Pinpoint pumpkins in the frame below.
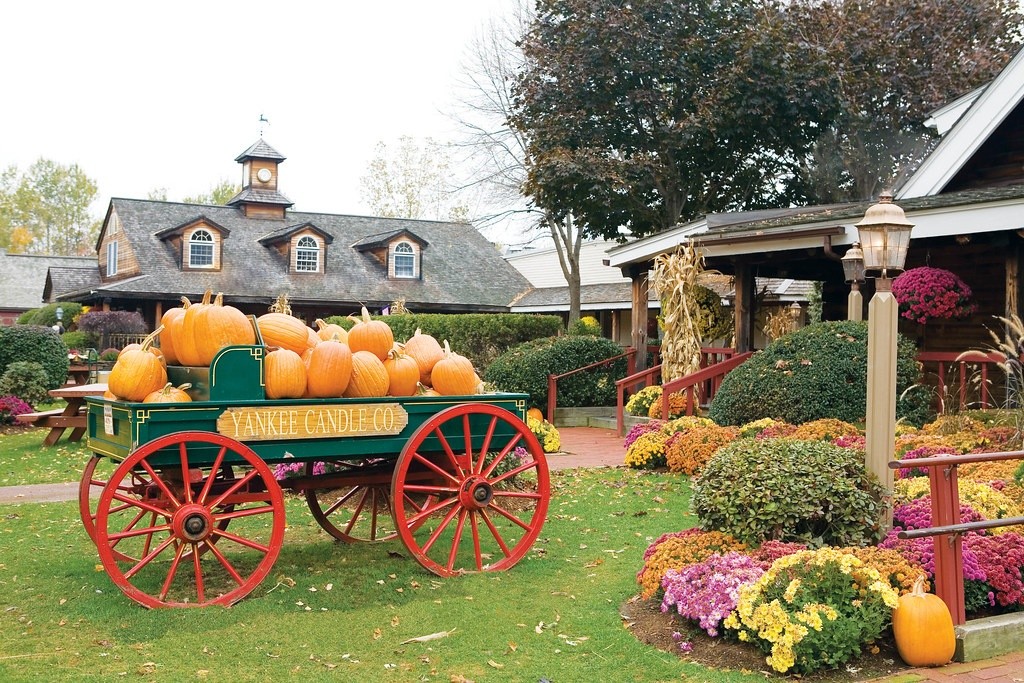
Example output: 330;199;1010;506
103;289;484;404
526;406;544;423
892;575;955;666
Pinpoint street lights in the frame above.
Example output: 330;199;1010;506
854;190;916;547
789;300;802;331
55;306;66;334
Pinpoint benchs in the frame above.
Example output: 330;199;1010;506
17;407;87;421
68;365;100;371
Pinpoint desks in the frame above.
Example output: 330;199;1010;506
71;360;105;384
32;384;110;448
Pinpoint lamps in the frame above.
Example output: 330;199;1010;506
55;306;65;321
853;192;914;292
840;241;867;285
789;300;802;322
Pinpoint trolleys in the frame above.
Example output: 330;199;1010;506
78;394;551;612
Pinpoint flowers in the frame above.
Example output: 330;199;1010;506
892;266;979;324
